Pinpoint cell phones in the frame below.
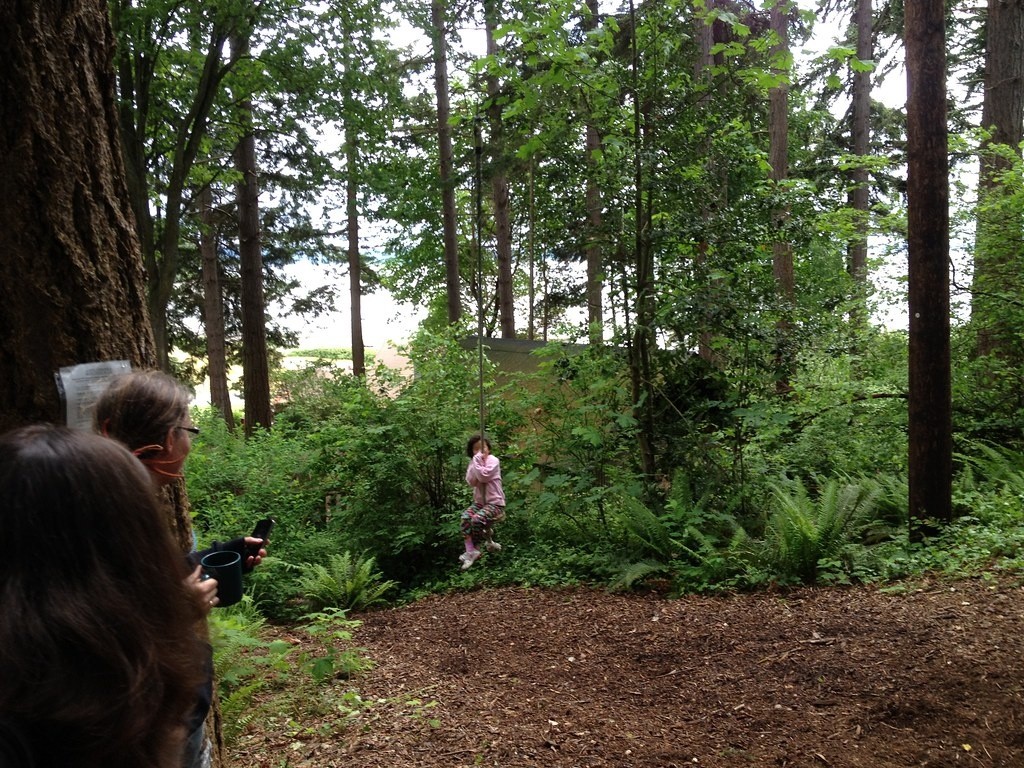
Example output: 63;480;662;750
245;519;275;561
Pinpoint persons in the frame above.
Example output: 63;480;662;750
90;368;271;768
459;436;506;569
0;426;209;768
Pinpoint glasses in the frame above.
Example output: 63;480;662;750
178;425;199;435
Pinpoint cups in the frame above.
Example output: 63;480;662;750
201;551;243;607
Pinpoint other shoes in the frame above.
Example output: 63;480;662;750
459;549;482;570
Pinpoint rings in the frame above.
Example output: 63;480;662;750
209;600;215;608
